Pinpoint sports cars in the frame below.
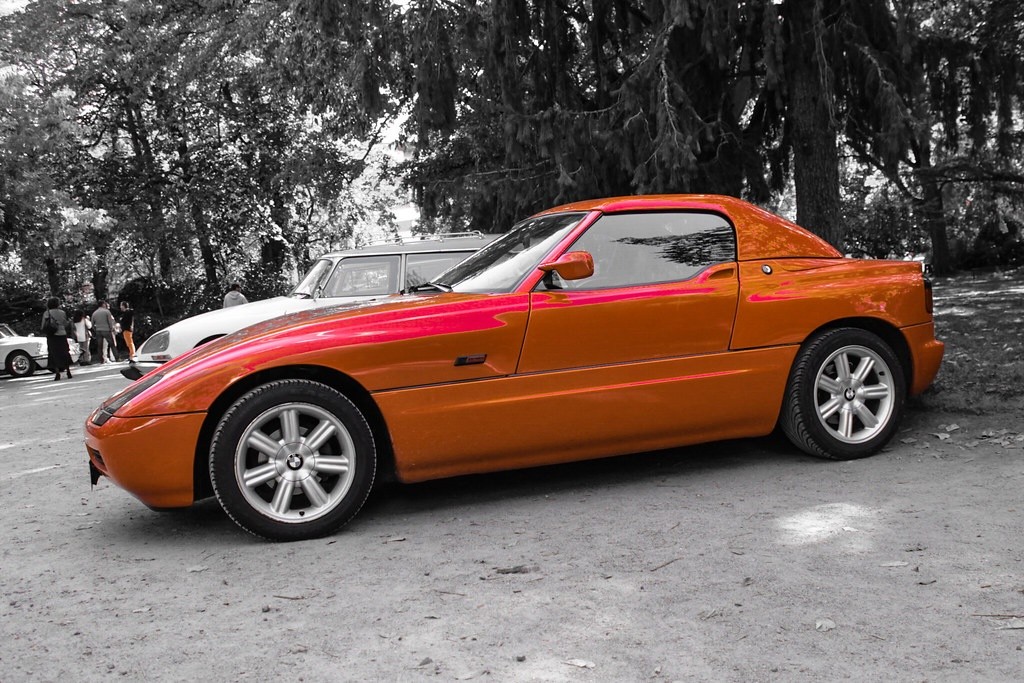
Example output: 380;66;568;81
83;190;945;543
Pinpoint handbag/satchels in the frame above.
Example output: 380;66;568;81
43;310;58;337
85;329;92;340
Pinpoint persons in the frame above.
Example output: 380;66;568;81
72;309;93;366
223;283;249;308
41;297;74;381
91;298;136;364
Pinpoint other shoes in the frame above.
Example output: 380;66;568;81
54;374;60;380
101;359;107;364
87;362;92;365
68;374;72;378
80;362;87;366
117;358;122;362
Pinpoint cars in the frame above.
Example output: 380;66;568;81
0;323;84;378
120;230;544;381
